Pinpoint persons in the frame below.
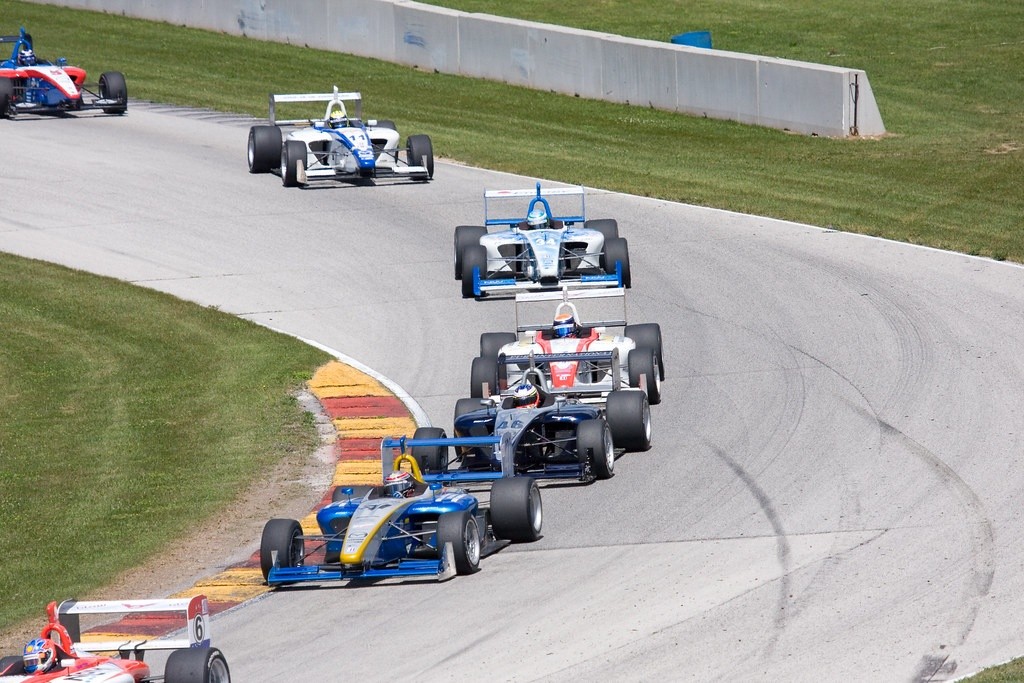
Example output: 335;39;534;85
526;209;552;230
552;313;578;338
20;636;59;675
513;384;540;410
18;48;40;67
382;470;421;498
328;111;352;128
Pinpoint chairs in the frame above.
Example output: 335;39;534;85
527;197;553;220
41;623;79;659
11;39;32;62
521;367;550;395
555;302;581;326
324;100;348;120
392;454;425;484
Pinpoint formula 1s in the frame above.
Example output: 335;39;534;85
0;27;128;117
453;180;632;298
411;349;652;481
247;85;434;187
470;285;665;405
260;433;543;587
0;594;231;683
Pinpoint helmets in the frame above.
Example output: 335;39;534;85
553;313;577;338
19;49;36;66
383;470;415;497
328;111;347;128
23;638;57;676
526;210;549;230
512;385;540;408
